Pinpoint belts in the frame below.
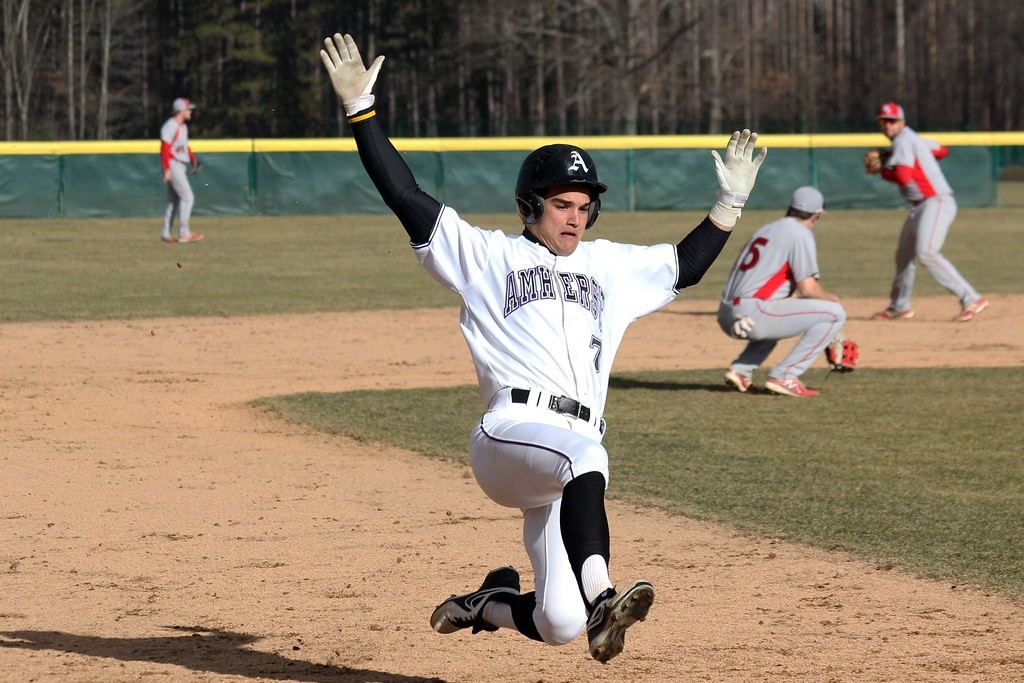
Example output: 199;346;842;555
511;389;604;435
913;197;929;205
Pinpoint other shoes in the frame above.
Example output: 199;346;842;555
871;307;916;320
161;234;178;243
953;297;989;321
177;232;204;243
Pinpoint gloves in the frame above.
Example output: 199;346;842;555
320;33;385;117
709;129;767;227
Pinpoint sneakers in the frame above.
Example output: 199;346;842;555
586;580;654;664
430;565;520;634
724;369;760;394
764;377;820;397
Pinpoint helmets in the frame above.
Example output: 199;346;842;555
515;144;608;230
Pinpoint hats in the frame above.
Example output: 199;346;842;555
172;97;197;112
790;186;825;213
874;102;905;120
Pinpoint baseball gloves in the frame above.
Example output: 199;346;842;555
189;160;204;174
863;148;886;174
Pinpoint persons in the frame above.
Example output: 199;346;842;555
863;100;989;322
160;98;204;243
318;32;769;666
717;186;859;401
